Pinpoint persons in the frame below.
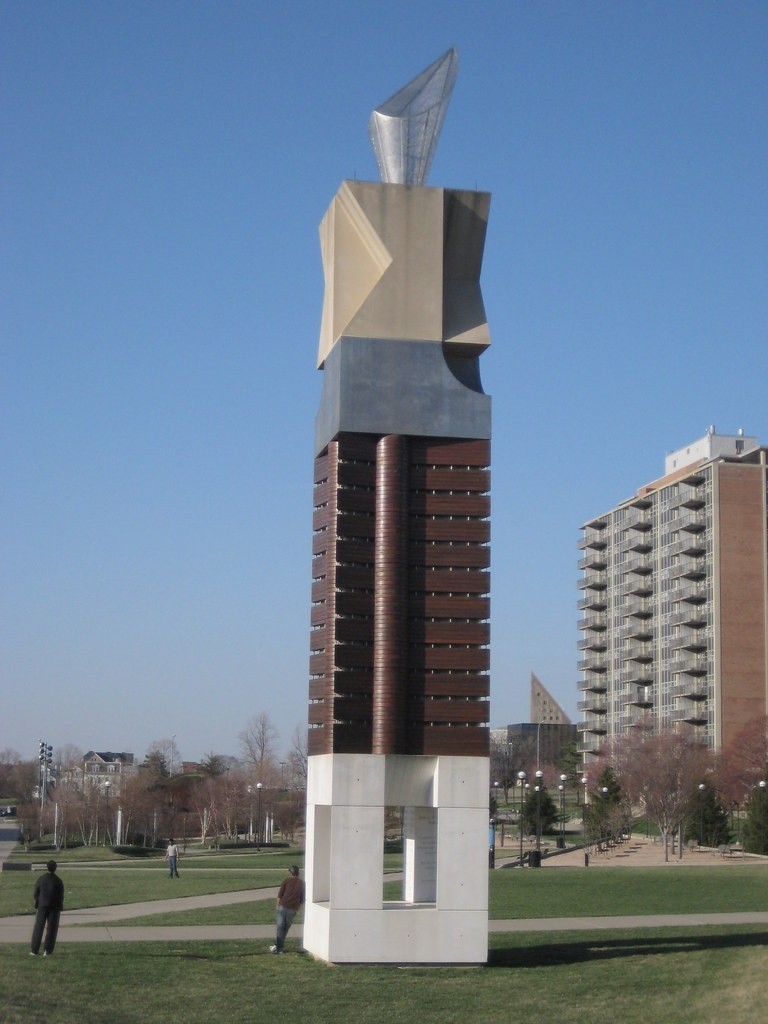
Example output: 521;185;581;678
29;860;64;958
270;865;305;954
164;839;180;878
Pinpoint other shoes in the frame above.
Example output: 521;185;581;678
29;952;39;956
44;951;50;956
269;942;283;955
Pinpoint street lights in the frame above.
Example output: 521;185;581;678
759;781;767;855
494;782;500;830
37;738;41;809
535;771;543;850
103;781;111;847
525;784;531;802
582;778;588;845
171;734;177;805
601;786;608;849
560;775;567;849
699;784;706;847
257;783;263;851
518;772;526;867
559;785;563;839
39;741;53;838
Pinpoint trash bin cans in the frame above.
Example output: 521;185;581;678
556;837;564;848
529;850;541;867
